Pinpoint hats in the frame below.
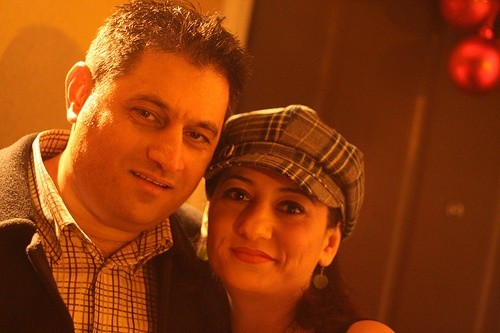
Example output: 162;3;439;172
203;104;365;240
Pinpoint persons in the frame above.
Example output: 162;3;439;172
0;1;253;333
199;105;393;333
